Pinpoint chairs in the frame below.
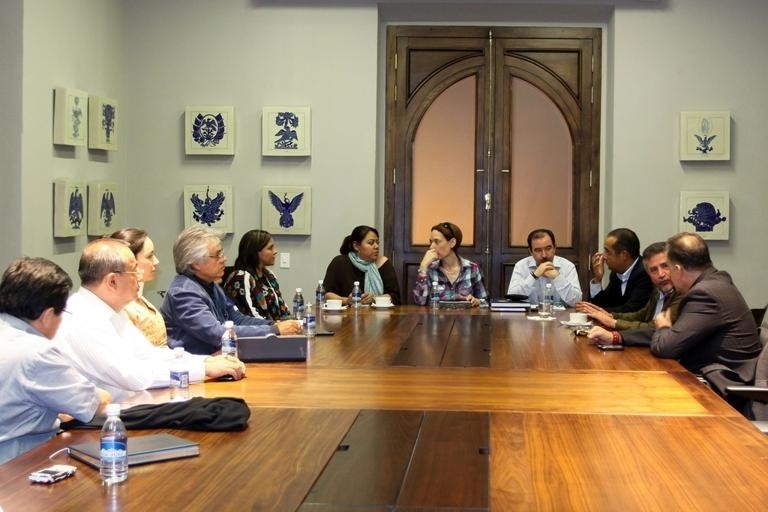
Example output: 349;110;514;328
217;265;236;289
158;291;168;297
698;302;768;436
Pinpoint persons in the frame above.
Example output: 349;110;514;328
575;242;683;331
413;222;487;306
160;224;303;355
52;240;246;403
506;229;584;308
100;230;168;404
1;258;112;464
588;227;655;312
324;226;402;306
586;232;764;423
225;228;291;321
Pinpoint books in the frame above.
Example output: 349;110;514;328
489;297;530;308
490;305;527;312
68;428;200;473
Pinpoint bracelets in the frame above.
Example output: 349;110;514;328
612;331;620;345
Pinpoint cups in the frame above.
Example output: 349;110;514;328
324;298;343;309
569;312;587;324
537;300;550;318
375;297;390;305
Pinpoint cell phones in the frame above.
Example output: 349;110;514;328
574;329;589;336
597;344;624;351
218;375;233;381
29;465;77;484
315;331;335;336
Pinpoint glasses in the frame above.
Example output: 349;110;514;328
112;267;144;281
198;248;225;259
440;220;456;234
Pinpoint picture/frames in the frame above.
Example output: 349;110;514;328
261;103;313;159
260;185;314;237
677;189;733;243
182;184;234;235
677;109;731;164
183;104;234;159
53;85;124;242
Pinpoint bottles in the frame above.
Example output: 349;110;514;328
98;403;129;483
351;280;361;312
168;346;190;401
544;284;554;317
292;280;327;339
221;320;238;357
430;282;440;310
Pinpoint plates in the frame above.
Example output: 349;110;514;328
566;322;591;327
371;305;395;309
322;306;347;311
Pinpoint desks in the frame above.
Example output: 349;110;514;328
0;301;768;511
